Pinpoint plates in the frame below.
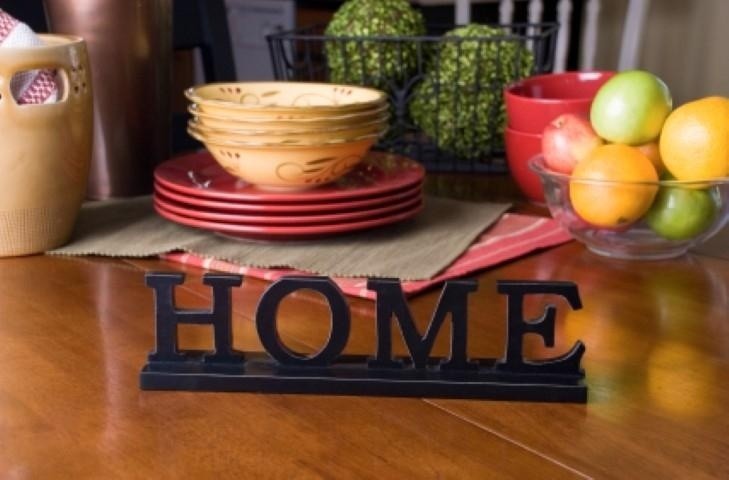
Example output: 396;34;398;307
154;151;430;239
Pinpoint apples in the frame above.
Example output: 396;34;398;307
542;112;604;176
635;139;667;177
590;71;674;144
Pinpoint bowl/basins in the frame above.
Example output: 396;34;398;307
182;77;391;190
505;69;624;130
499;131;590;202
534;149;728;261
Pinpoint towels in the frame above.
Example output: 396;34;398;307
154;212;578;307
53;196;513;283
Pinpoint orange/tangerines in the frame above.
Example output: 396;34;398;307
637;255;712;337
646;171;716;241
